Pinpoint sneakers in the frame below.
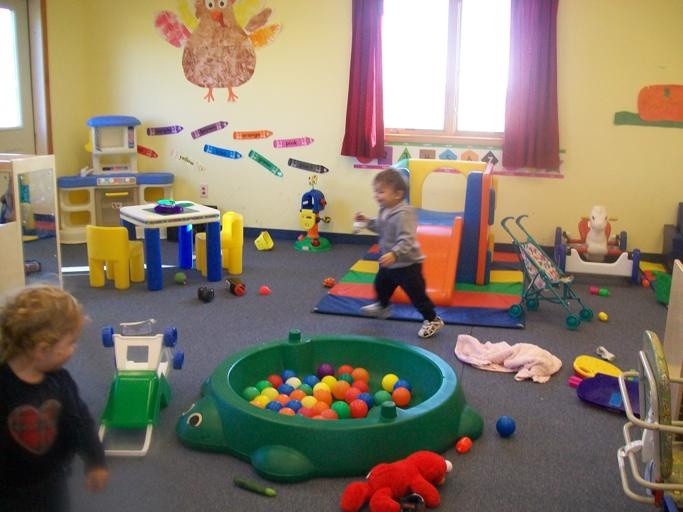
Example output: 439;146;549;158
359;300;392;320
417;315;445;338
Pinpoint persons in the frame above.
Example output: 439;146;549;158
353;169;445;338
0;285;108;512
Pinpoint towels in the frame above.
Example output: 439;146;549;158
455;334;562;384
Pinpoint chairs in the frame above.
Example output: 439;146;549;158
196;212;243;275
87;225;144;289
618;329;683;512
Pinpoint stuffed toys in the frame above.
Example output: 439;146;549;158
341;449;453;511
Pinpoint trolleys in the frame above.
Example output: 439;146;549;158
500;212;595;331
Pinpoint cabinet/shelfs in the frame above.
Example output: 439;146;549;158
0;153;58;292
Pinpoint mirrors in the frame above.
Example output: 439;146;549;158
18;169;60;285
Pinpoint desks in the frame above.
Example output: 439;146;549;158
119;200;222;291
